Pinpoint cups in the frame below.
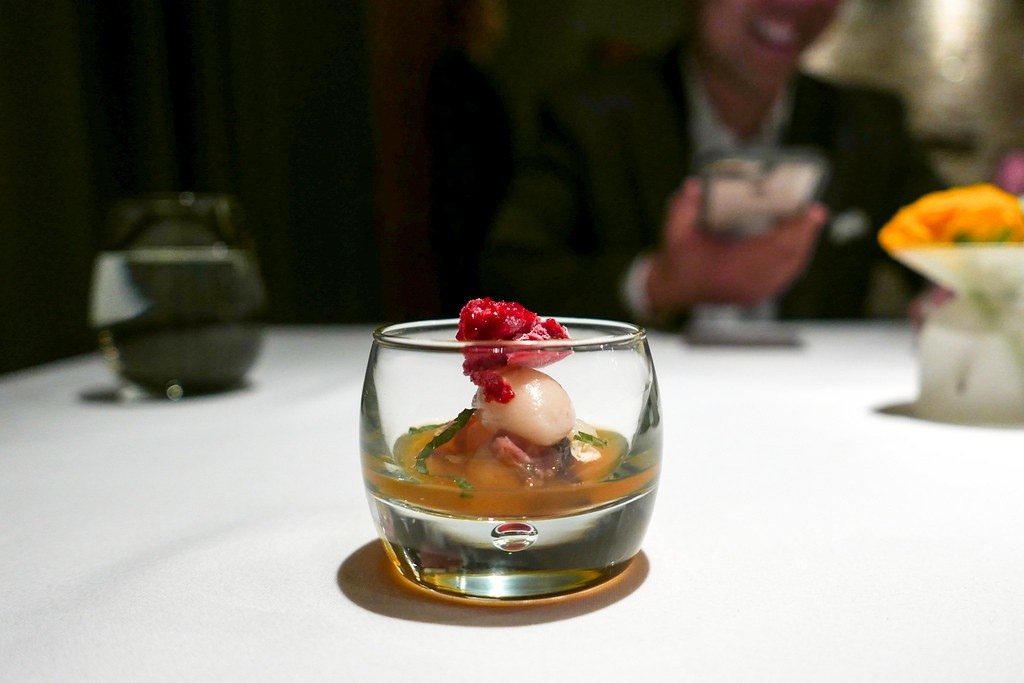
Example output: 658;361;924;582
361;315;661;604
91;198;270;395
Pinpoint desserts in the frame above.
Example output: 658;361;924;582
449;297;576;445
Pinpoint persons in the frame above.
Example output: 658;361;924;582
480;0;949;334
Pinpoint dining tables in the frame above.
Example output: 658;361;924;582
0;321;1024;683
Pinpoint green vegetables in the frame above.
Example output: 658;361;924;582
407;408;606;499
